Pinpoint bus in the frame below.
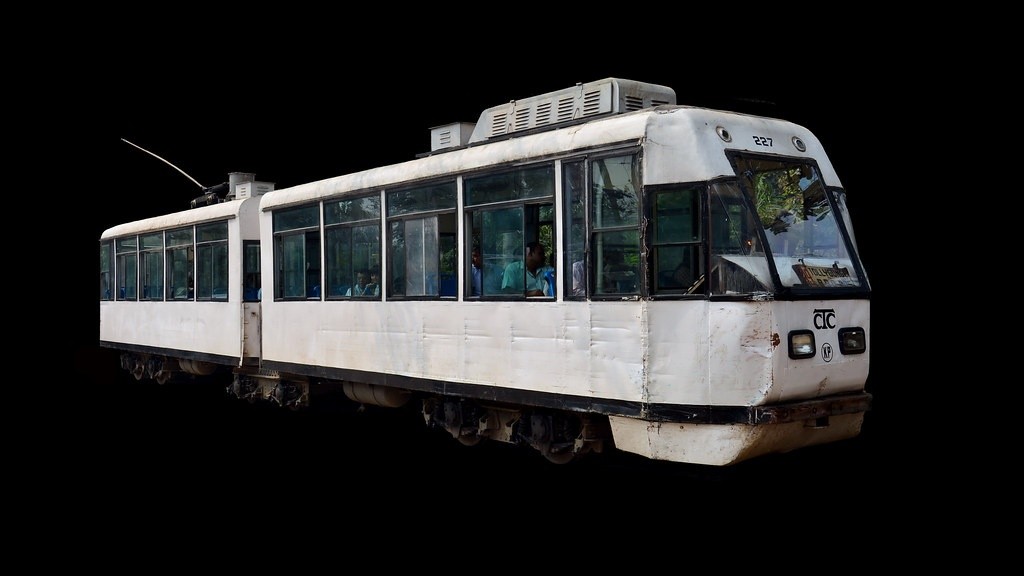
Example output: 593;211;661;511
96;76;874;467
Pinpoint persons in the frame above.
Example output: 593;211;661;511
283;228;699;296
772;213;800;257
104;253;225;299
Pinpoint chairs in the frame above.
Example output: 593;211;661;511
213;262;641;300
